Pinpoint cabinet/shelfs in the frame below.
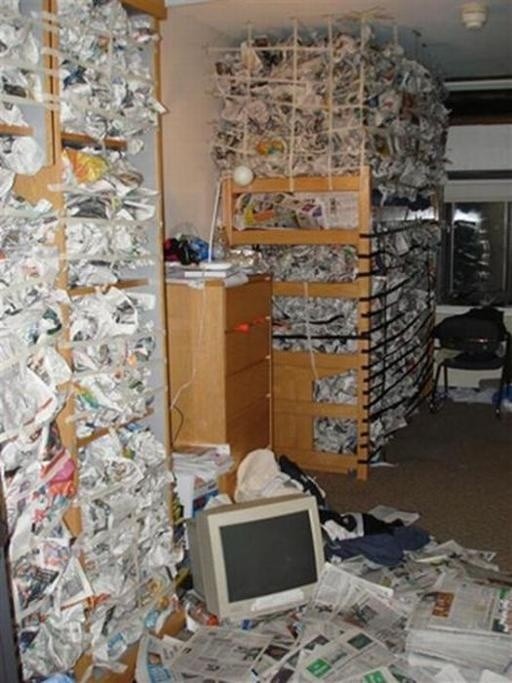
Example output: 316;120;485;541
0;0;189;683
165;270;274;502
221;163;446;482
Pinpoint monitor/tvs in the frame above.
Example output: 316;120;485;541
185;495;327;622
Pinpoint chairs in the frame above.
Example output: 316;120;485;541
428;306;511;422
